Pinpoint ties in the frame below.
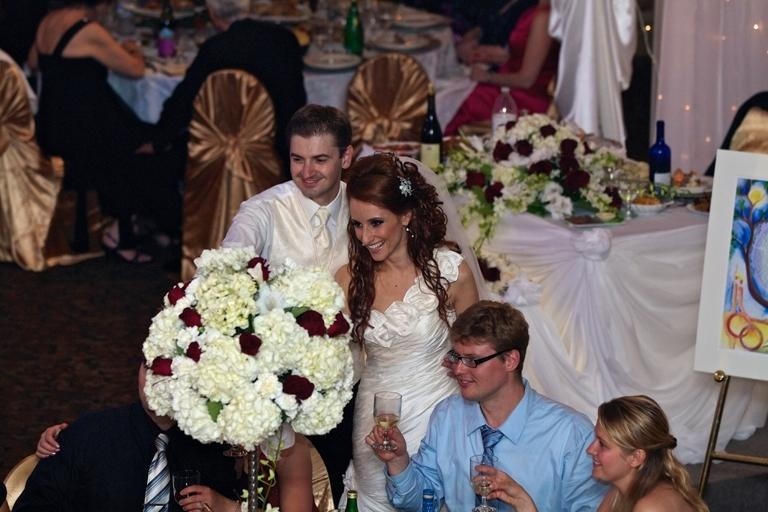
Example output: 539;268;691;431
144;432;171;511
314;208;332;255
475;425;503;511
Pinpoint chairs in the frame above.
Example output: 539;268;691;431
339;49;428;164
1;53;109;274
173;64;288;279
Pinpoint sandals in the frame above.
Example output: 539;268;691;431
101;228;154;264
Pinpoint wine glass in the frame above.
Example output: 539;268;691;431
170;469;201;510
468;454;502;512
374;390;403;451
614;171;643;220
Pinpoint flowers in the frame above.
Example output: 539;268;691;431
132;244;362;459
436;107;657;295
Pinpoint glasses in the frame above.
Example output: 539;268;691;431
445;349;513;368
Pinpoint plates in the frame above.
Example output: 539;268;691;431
300;53;362;71
370;30;432;52
682;200;711;215
244;4;308;21
390;11;444;29
119;1;195;18
625;202;673;215
662;175;716;196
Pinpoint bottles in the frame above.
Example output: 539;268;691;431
345;2;364;55
421;79;444;172
344;491;359;511
490;86;519;147
159;2;177;57
648;119;671;187
421;488;435;511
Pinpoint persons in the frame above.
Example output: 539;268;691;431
2;2;709;511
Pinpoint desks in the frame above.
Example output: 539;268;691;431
82;3;456;177
420;169;768;480
